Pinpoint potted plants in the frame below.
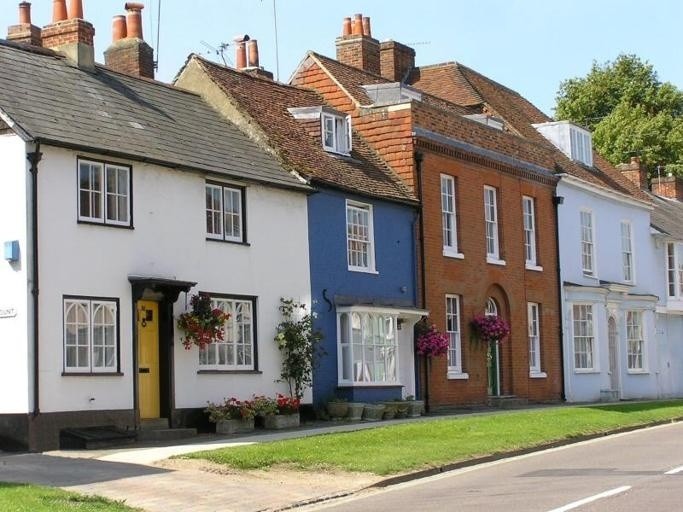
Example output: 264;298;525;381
325;395;424;422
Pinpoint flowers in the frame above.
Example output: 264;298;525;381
412;317;450;361
202;391;302;423
172;291;231;351
271;294;327;413
468;314;511;343
